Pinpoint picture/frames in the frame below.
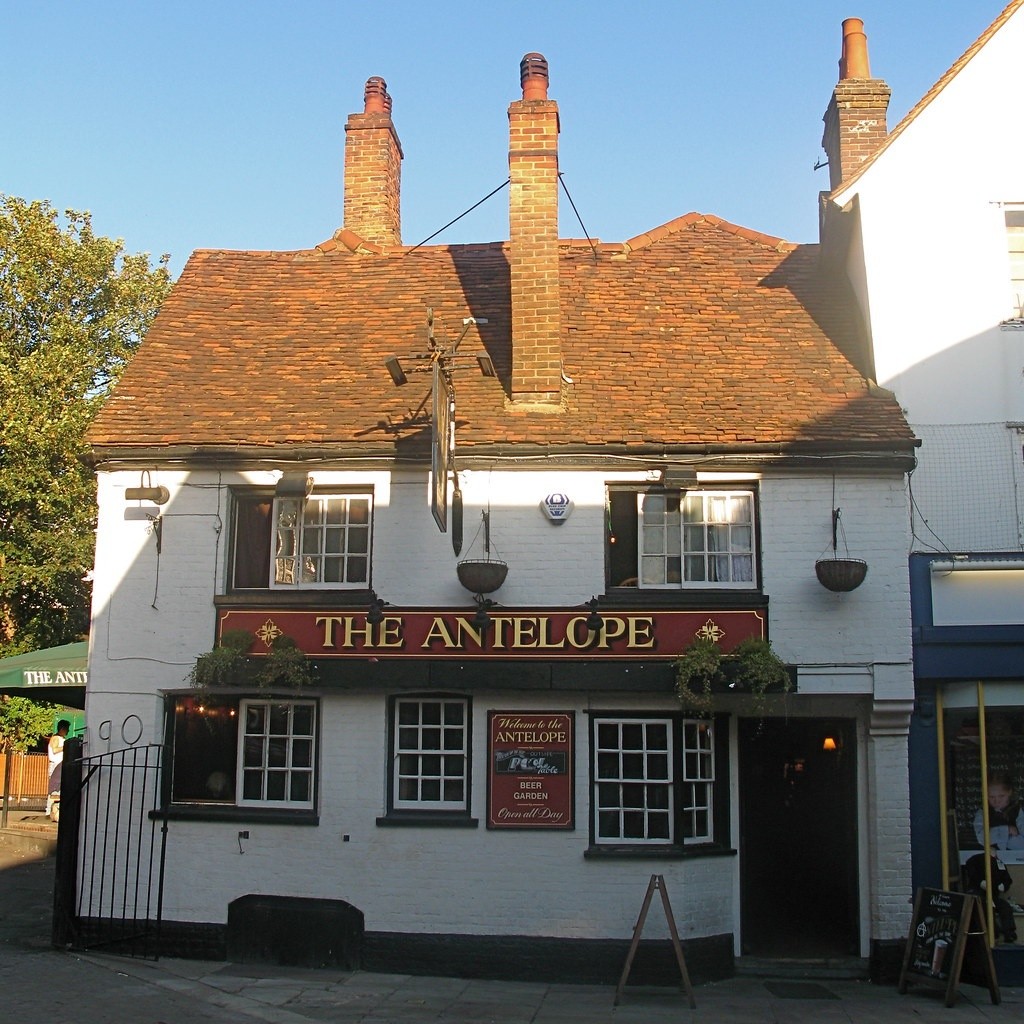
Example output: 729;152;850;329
487;709;577;830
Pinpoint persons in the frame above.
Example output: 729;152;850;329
974;775;1024;851
46;720;71;822
966;843;1018;946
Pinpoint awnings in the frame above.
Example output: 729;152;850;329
0;641;89;711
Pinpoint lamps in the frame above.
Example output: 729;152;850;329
125;469;170;505
367;590;390;623
662;465;700;491
585;595;604;630
275;470;314;500
474;593;498;629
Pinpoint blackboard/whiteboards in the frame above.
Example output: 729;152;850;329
903;887;974;991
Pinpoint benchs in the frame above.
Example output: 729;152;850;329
228;893;367;972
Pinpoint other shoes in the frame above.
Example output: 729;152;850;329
1013;905;1024;912
